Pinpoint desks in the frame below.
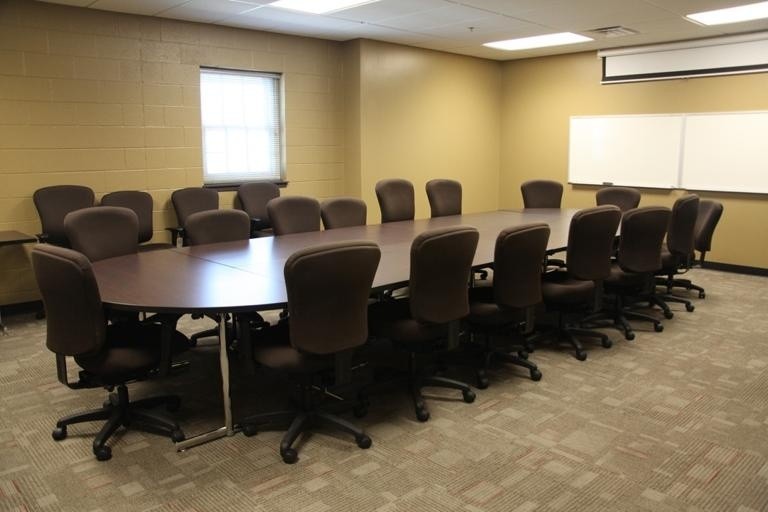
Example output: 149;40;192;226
91;208;624;452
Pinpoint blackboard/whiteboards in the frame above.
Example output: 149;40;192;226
679;111;768;193
566;113;685;190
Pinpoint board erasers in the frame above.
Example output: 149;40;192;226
603;182;613;185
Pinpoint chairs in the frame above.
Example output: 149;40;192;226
577;208;672;340
655;201;722;298
321;199;367;228
377;182;414;223
426;181;462;217
432;224;550;380
184;208;268;343
64;205;190;373
101;191;174;252
521;181;562;209
266;196;321;236
32;243;191;460
596;187;640;211
237;182;280;236
624;194;698;319
353;228;479;421
524;208;622;361
241;244;380;463
34;186;94;249
164;187;218;247
